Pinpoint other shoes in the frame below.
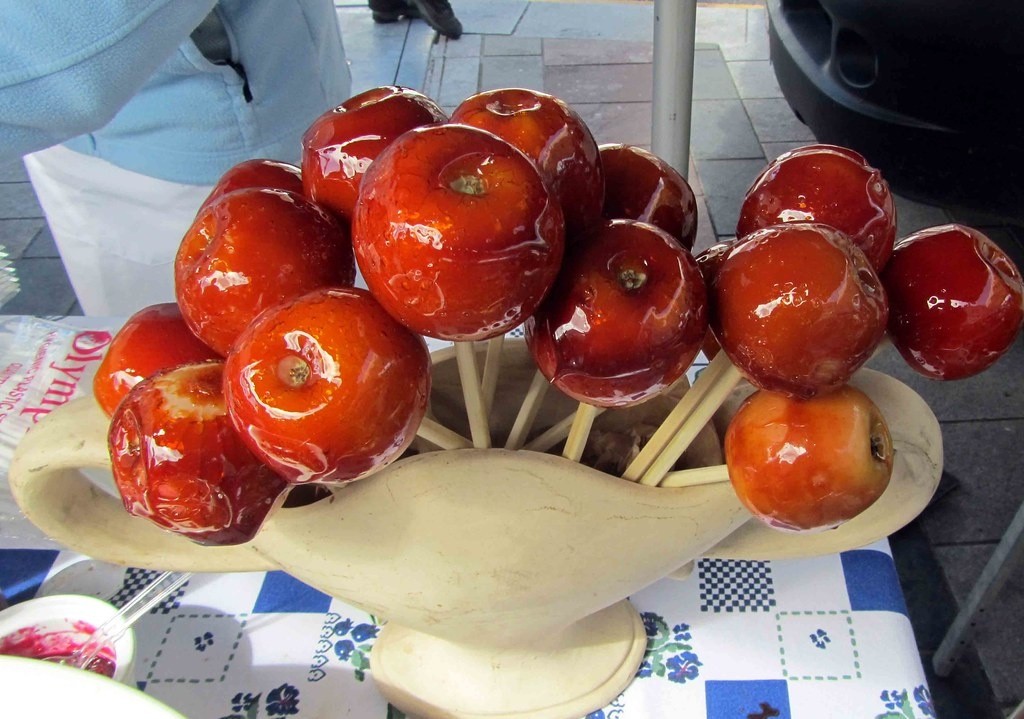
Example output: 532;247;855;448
368;0;421;23
413;0;462;40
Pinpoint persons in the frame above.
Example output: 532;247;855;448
0;0;355;320
369;0;462;40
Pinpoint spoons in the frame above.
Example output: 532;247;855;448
41;570;197;671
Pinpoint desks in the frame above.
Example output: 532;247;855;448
0;313;940;719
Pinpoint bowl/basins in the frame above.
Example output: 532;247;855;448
0;592;135;683
